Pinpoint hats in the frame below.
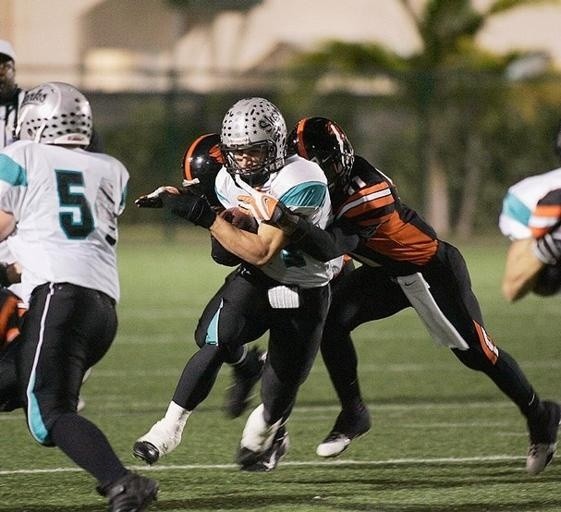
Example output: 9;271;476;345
0;37;15;62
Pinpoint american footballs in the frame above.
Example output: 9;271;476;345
220;207;258;233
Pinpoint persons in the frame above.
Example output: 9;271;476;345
499;167;561;304
0;38;26;147
134;98;344;470
0;81;159;512
210;117;561;474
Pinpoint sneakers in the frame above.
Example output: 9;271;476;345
133;418;186;462
522;397;560;475
239;425;290;473
236;401;279;467
225;350;269;420
315;405;372;457
99;469;161;512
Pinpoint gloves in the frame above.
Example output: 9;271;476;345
135;186;174;211
237;187;280;225
162;189;216;229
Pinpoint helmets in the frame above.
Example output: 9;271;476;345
14;81;94;149
219;93;288;178
181;131;224;191
286;115;356;191
